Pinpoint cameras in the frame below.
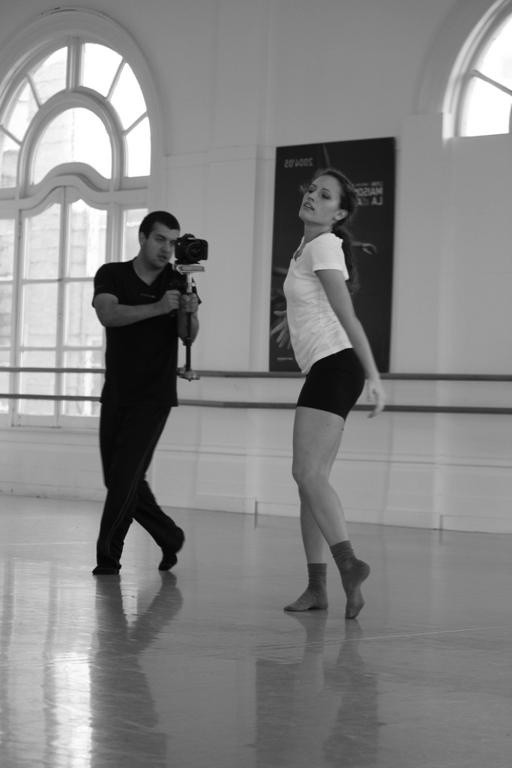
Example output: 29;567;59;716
174;233;209;263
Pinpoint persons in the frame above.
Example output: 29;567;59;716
267;168;386;621
251;604;380;768
87;563;184;768
91;209;204;576
